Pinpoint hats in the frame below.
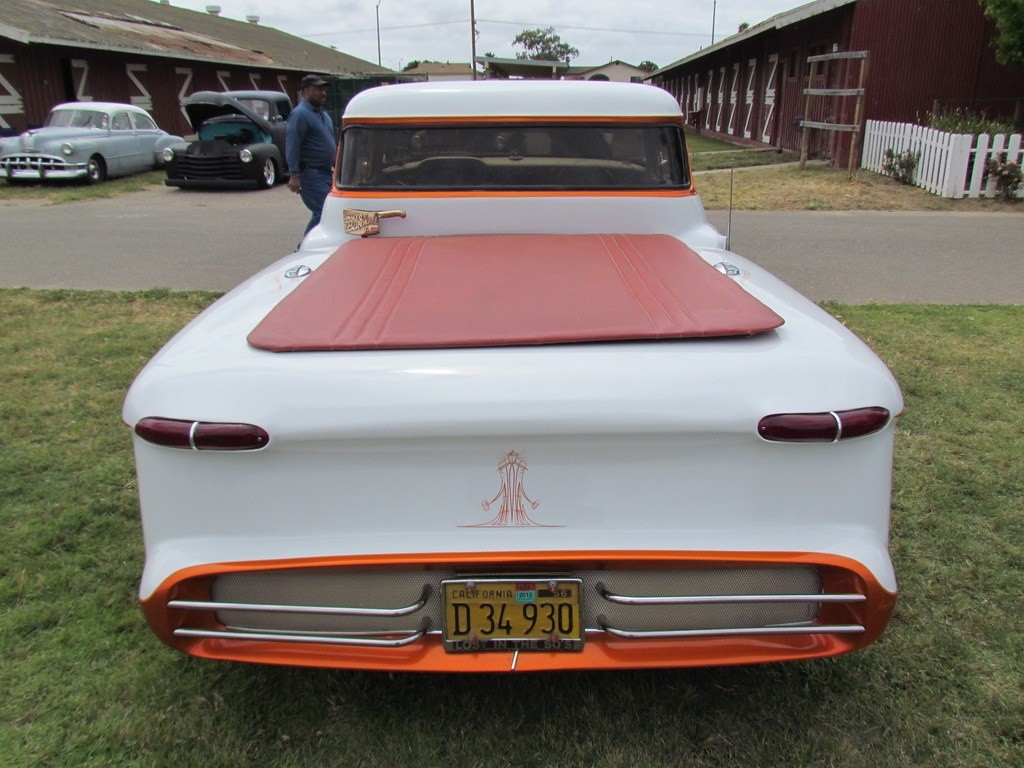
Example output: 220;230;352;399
300;74;330;90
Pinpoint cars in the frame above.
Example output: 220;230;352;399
163;87;294;189
0;102;184;184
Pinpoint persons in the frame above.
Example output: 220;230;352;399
285;74;338;254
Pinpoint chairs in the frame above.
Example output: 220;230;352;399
409;158;489;189
545;153;614;189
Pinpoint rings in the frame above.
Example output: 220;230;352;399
299;186;301;190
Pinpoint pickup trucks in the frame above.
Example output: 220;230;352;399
123;81;905;674
